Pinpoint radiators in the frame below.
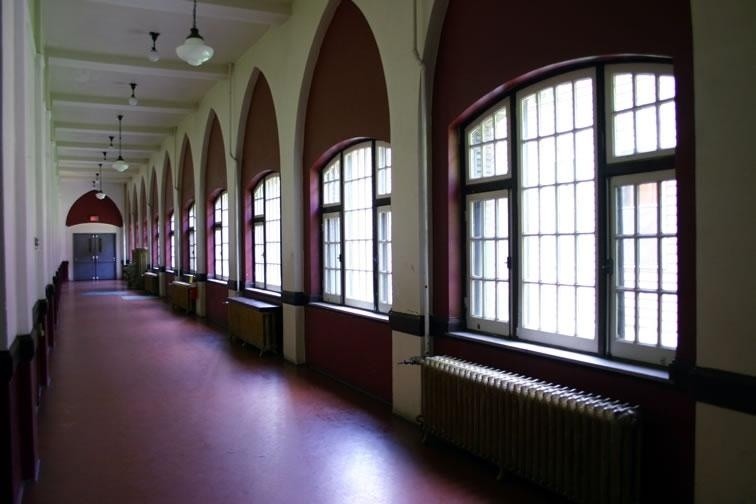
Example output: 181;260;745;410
419;355;640;504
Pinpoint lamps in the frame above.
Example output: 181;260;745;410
95;163;106;200
111;114;129;173
175;0;215;67
